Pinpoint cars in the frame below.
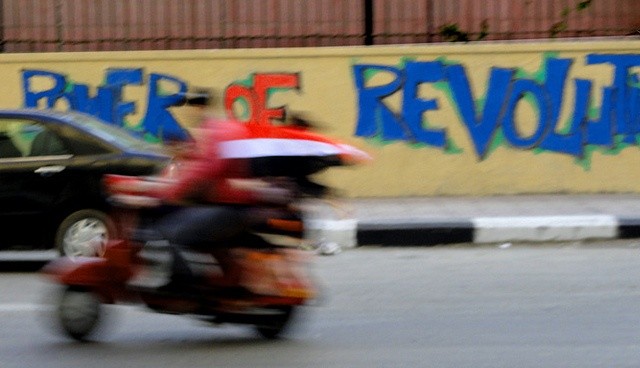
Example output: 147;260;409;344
0;107;182;266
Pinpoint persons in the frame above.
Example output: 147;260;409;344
125;86;267;287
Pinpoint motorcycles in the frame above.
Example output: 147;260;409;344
37;115;372;341
